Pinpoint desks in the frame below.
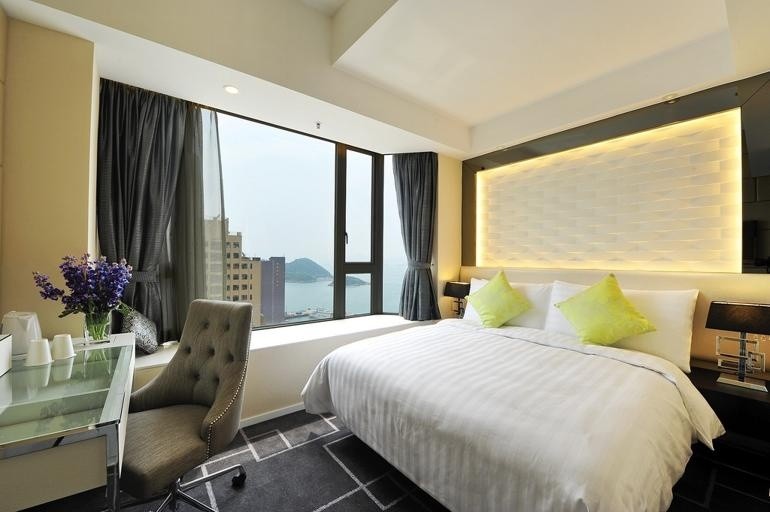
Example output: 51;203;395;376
0;331;137;511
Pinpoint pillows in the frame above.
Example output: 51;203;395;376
463;278;551;329
554;274;655;345
122;307;158;353
545;281;699;373
465;270;531;328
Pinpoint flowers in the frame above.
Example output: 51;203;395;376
32;250;133;318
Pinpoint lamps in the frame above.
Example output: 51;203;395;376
444;281;470;316
706;301;770;394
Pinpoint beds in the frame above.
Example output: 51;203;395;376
300;320;726;512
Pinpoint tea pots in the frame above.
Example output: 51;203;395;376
2;311;43;361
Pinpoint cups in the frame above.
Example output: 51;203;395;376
52;358;74;382
24;338;54;367
25;364;52;388
53;334;77;360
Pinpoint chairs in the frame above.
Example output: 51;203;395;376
121;299;254;512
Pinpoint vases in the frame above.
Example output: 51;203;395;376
84;311;111;343
